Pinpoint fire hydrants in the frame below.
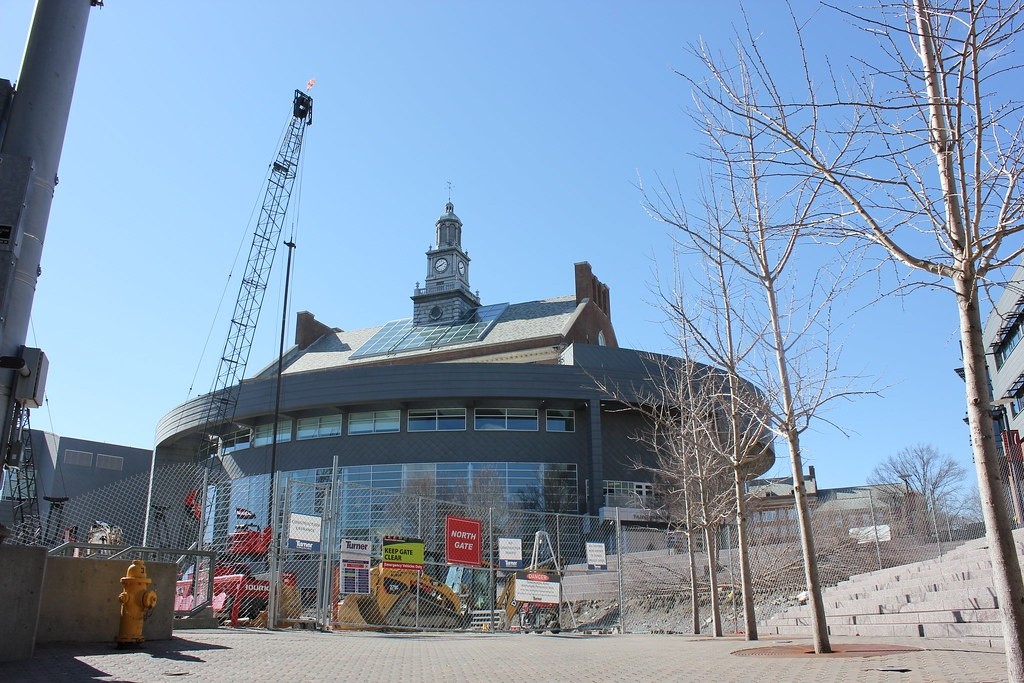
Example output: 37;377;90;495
112;556;159;649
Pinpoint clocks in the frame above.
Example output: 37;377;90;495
458;261;465;275
434;258;448;272
